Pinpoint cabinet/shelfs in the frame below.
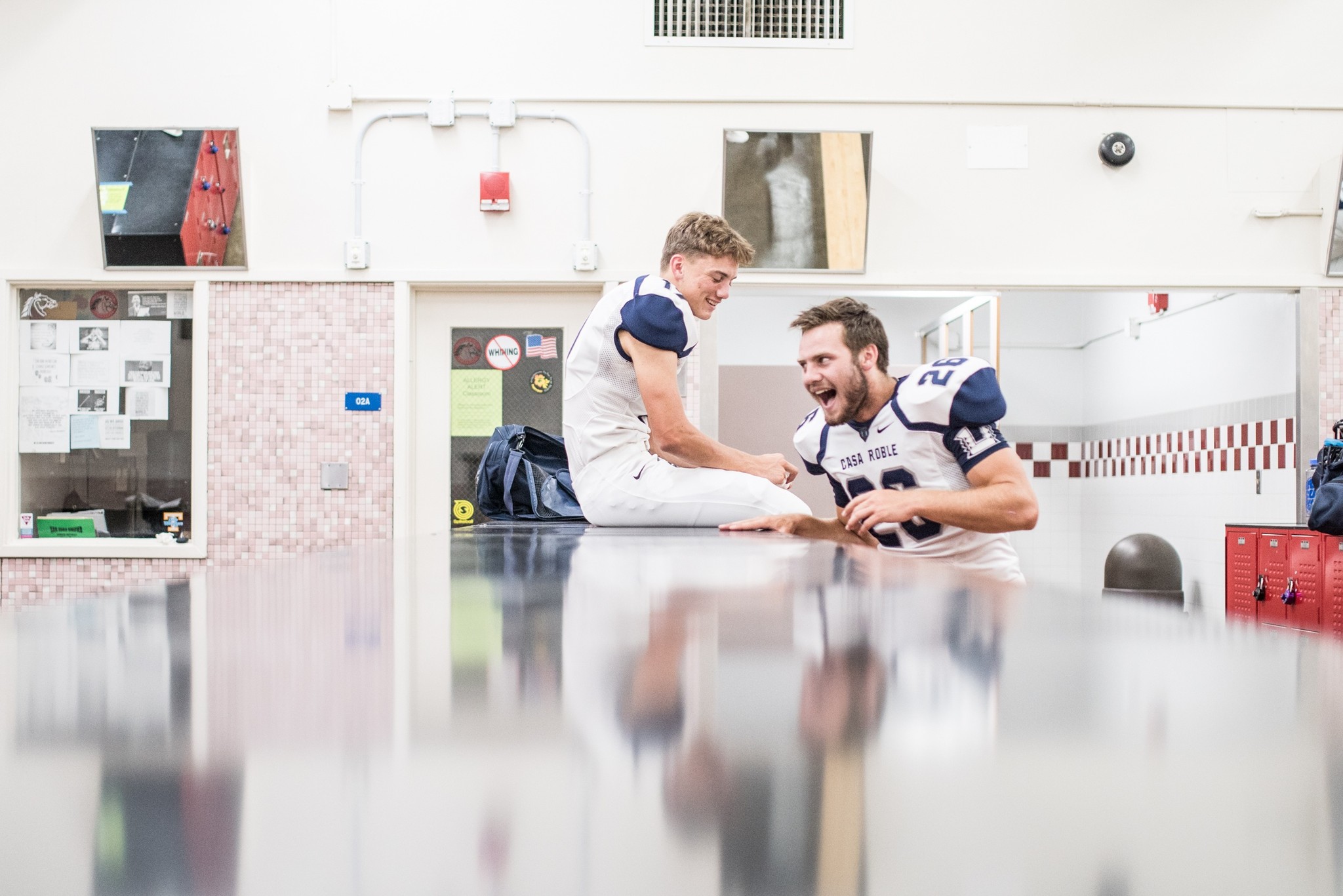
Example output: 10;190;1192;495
94;130;240;266
1223;523;1343;647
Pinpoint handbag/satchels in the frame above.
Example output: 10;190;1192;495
475;424;586;521
1308;460;1343;536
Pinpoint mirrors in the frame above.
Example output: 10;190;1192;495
721;128;873;274
91;127;248;272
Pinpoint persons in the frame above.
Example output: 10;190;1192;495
754;137;816;268
716;297;1039;583
80;328;108;351
138;361;152;371
561;529;1004;834
562;213;813;527
128;295;150;316
